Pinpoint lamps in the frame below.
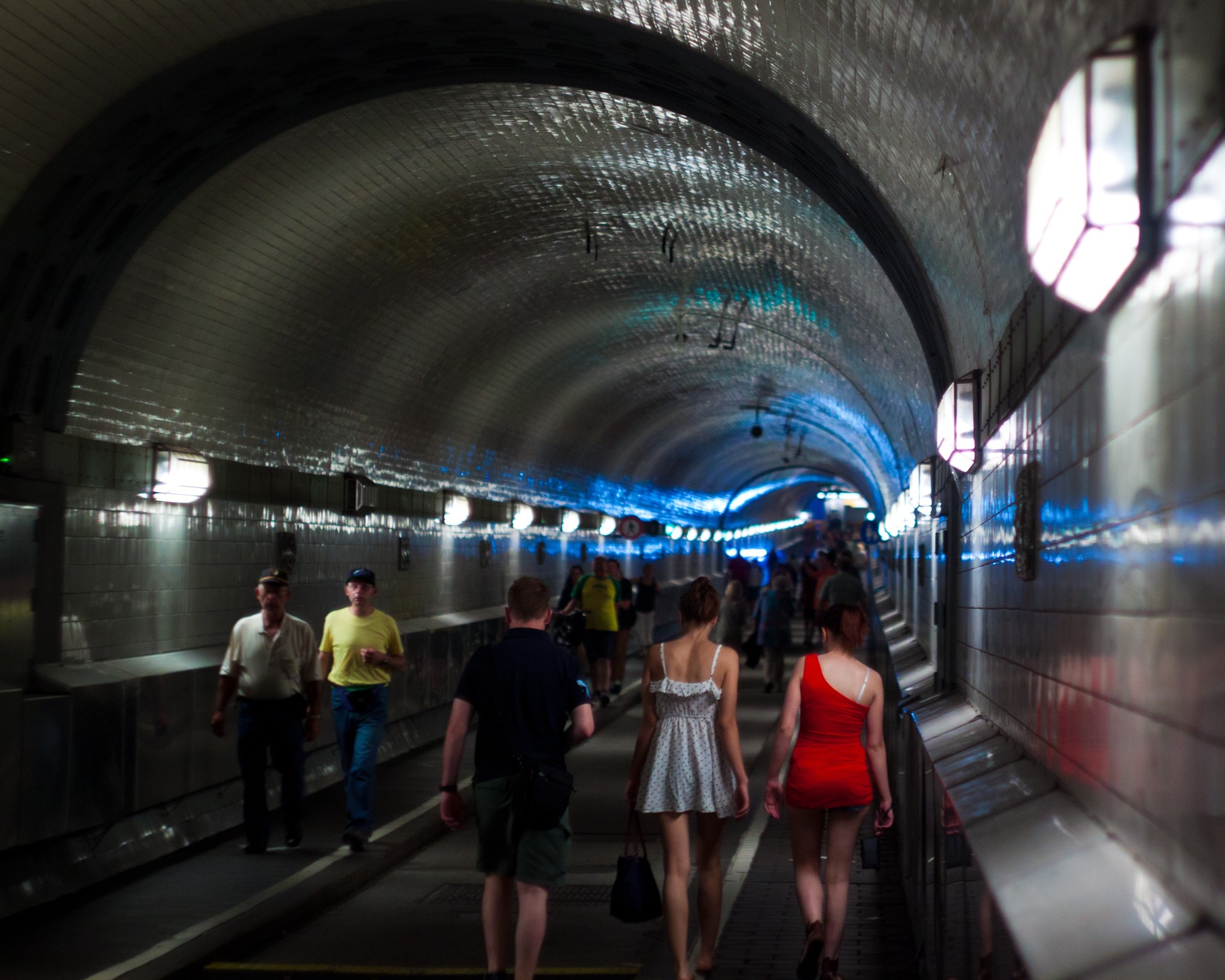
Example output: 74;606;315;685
151;442;213;505
343;472;379;517
1026;0;1158;315
909;455;937;517
935;369;982;475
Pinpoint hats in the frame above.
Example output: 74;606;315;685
258;569;290;588
347;568;377;585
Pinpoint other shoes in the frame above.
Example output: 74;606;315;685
342;828;368;852
285;817;302;847
245;830;267;854
600;691;611;707
795;928;822;980
610;676;623;695
765;681;776;693
818;957;844;980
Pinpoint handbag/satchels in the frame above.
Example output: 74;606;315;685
609;800;663;923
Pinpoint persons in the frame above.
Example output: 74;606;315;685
715;581;747;651
763;603;894;980
751;575;797;693
785;545;861;647
747;559;762;604
210;564;319;855
440;577;595;980
318;567;406;854
555;558;633;708
625;576;750;980
632;564;661;651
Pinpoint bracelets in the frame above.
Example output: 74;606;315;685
440;785;457;792
